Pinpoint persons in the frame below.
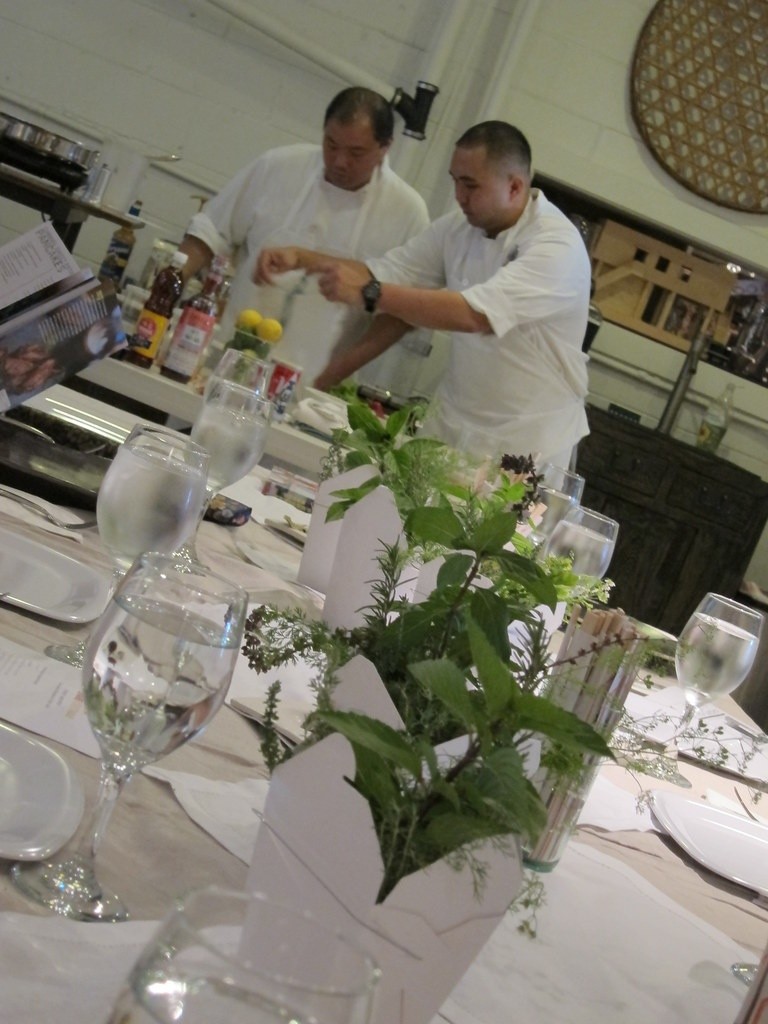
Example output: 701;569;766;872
249;120;594;497
171;85;430;391
0;316;123;398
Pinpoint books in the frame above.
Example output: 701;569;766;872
0;220;95;321
1;276;129;416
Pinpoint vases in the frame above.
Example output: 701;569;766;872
249;731;522;1024
332;650;540;780
319;483;420;637
297;457;387;594
411;555;565;653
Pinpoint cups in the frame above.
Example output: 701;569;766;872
224;329;272;358
515;463;584;545
267;357;305;414
106;888;379;1024
517;679;623;873
545;505;619;598
202;349;276;414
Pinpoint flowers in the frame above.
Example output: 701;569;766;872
318;394;615;618
243;551;721;744
242;619;767;941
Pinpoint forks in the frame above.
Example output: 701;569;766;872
0;489;97;531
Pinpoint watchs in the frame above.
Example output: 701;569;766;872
362;278;381;316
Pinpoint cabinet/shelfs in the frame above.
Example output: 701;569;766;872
577;403;768;637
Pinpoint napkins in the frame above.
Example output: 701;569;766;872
141;764;759;1024
0;912;244;1023
579;777;668;836
705;783;768;827
220;479;312;529
158;591;326;751
0;484;85;546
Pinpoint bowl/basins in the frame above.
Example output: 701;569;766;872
302;385;350;413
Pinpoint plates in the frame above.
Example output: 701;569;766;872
649;786;768;896
0;726;86;861
639;620;686;661
0;529;117;623
620;686;768;785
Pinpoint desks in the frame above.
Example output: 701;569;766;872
0;385;768;1024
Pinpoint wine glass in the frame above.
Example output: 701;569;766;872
732;963;760;986
10;551;249;923
42;425;211;664
175;382;273;572
660;592;763;747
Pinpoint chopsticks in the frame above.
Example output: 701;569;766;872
523;602;646;863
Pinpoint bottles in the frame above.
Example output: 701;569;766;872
99;199;142;298
127;251;189;369
214;281;231;323
697;383;737;451
160;257;229;384
